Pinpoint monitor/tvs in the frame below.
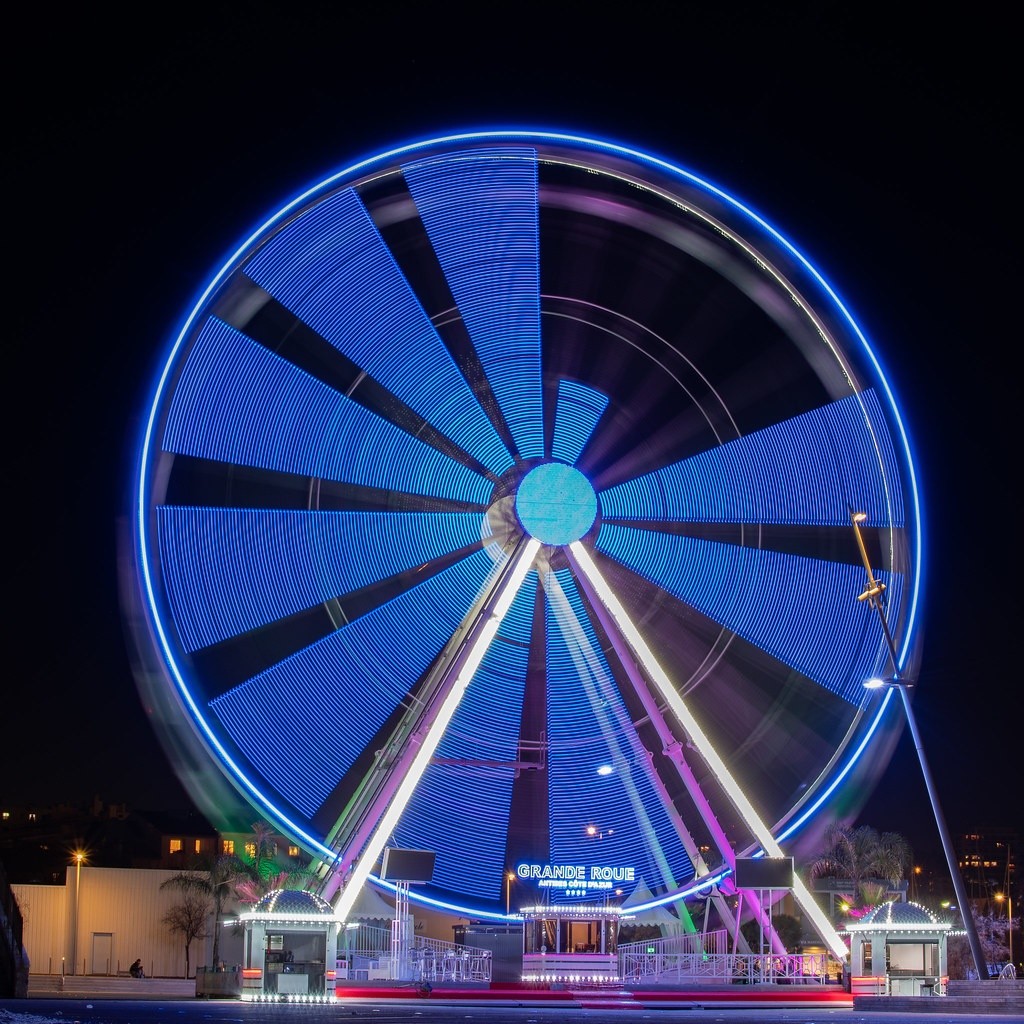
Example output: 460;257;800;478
735;856;795;890
383;849;436;883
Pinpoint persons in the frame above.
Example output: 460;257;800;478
841;954;851;993
789;944;805;974
129;959;144;979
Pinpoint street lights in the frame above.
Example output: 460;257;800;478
506;875;515;933
72;855;83;976
911;867;921;901
996;895;1013;964
847;501;990;981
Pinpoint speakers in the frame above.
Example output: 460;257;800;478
420;982;432;992
550;983;563;990
